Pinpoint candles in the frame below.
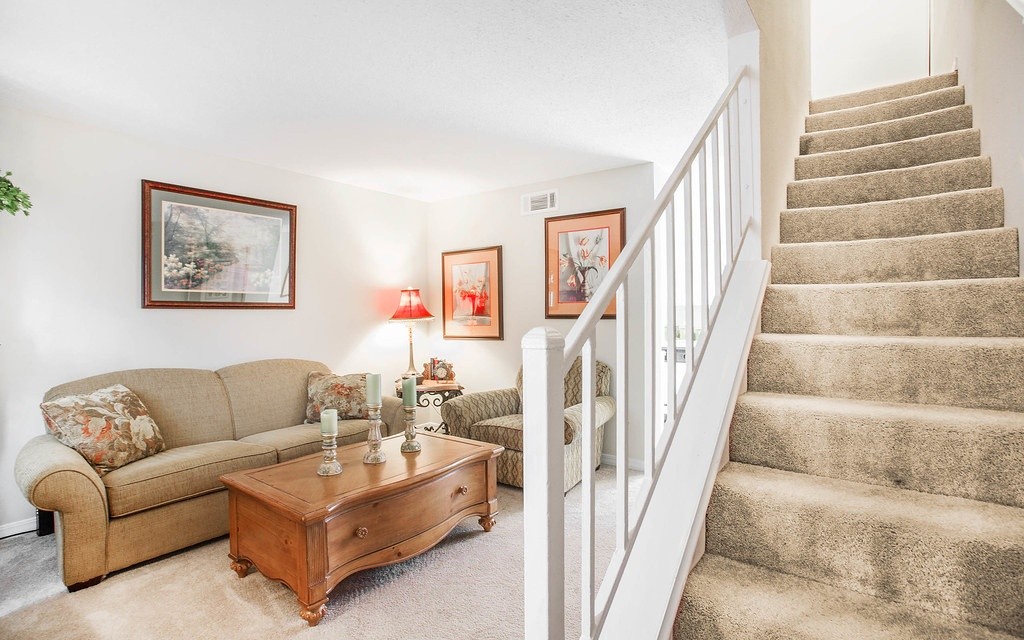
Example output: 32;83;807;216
366;374;381;404
401;375;417;407
320;409;337;436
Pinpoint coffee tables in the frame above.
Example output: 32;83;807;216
218;430;505;626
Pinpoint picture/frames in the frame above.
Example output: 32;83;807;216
142;179;297;310
544;207;626;320
441;246;503;340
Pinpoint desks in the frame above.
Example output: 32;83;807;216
395;384;464;435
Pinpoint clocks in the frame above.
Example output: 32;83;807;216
433;362;448;379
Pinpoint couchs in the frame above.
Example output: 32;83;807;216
440;353;616;497
14;358;406;592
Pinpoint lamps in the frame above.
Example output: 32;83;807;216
388;286;436;376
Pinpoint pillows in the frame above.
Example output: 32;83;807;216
304;370;369;419
40;384;165;476
